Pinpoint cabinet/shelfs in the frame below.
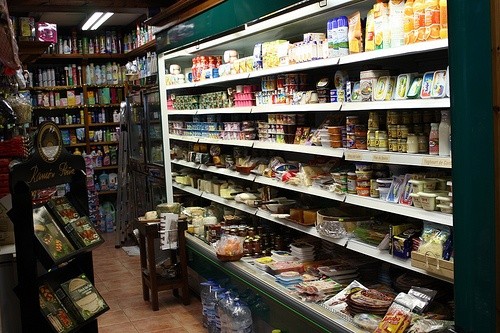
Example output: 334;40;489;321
8;122;110;333
17;40;156;195
156;0;500;333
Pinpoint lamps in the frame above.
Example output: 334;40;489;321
81;11;114;30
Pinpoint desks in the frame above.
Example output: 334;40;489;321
137;217;191;311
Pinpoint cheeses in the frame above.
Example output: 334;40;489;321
175;168;317;235
145;202;184;220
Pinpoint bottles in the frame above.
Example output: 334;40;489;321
192;55;222;83
272;329;281;333
30;19;163;232
201;279;255;333
429;110;452;159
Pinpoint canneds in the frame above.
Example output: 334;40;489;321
168;110;305;143
340;163;385;198
192;55;223;82
326;16;349;57
224;226;281;257
173;72;338;110
345;109;429;154
